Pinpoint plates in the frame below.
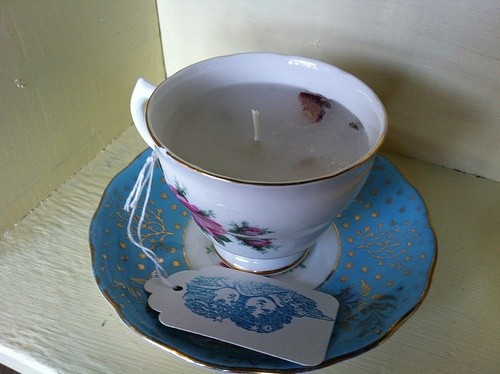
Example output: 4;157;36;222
88;146;438;374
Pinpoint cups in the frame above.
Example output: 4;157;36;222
131;52;388;272
